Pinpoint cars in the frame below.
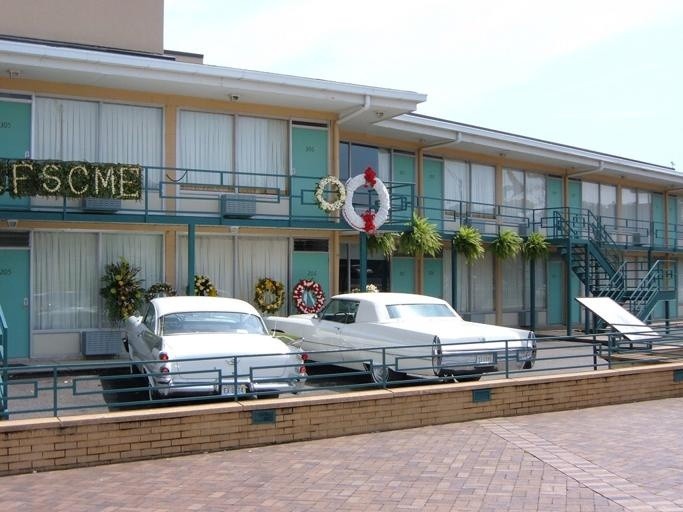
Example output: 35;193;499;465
33;291;100;329
123;295;308;399
261;292;536;389
